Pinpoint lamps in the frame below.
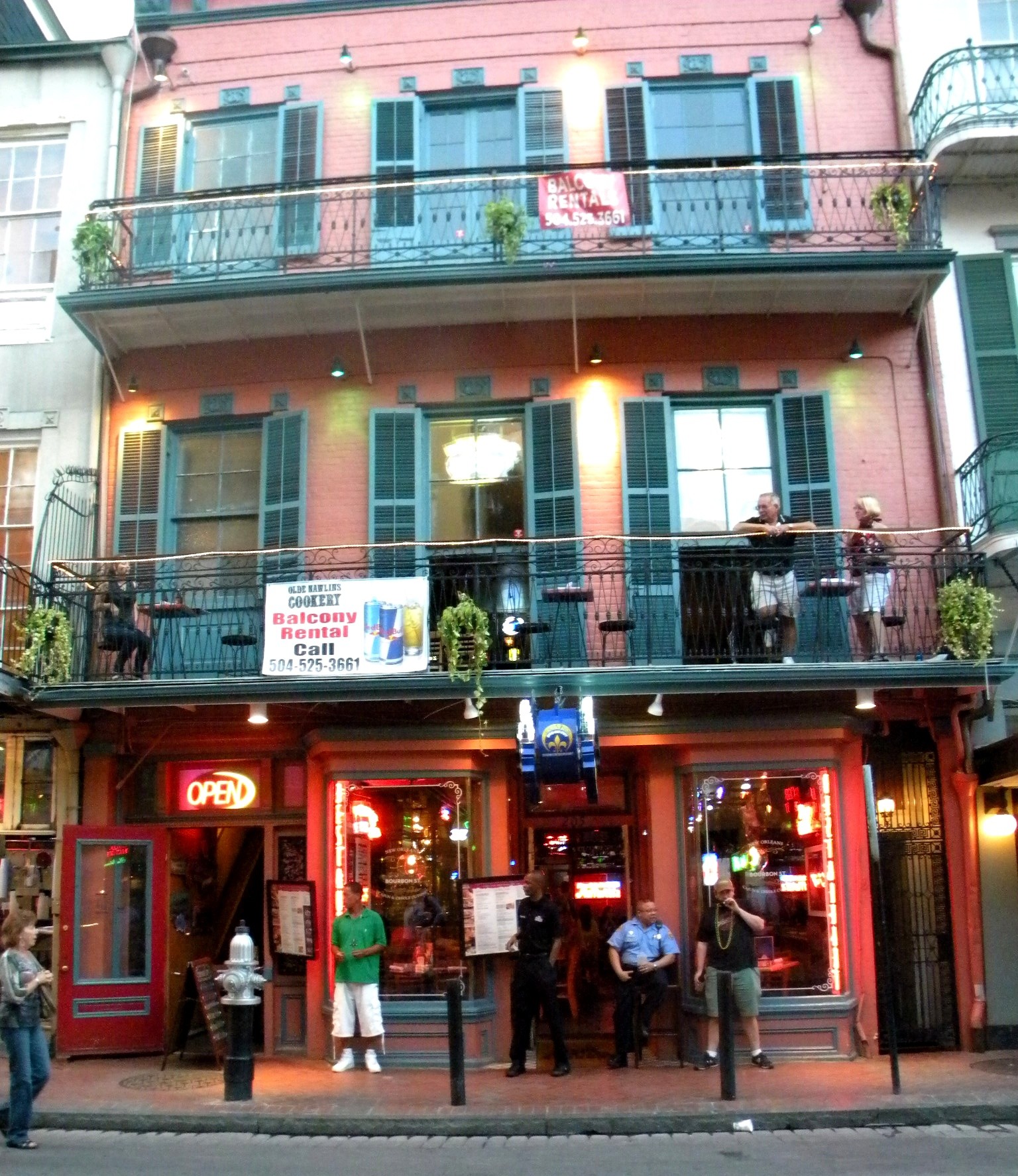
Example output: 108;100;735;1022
464;698;479;719
340;45;354;71
330;357;345;381
154;58;175;90
981;789;1018;839
647;694;663;716
805;15;823;46
590;346;602;363
127;377;138;392
849;339;863;358
248;703;269;722
854;689;876;708
573;27;588;55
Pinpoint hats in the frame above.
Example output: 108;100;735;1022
715;880;732;892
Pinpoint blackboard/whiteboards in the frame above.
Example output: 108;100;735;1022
165;957;229;1057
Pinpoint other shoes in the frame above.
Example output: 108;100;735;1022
112;675;133;681
764;629;776;647
863;657;889;662
783;657;794;664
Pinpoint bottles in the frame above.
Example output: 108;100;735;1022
174;589;183;604
692;970;706;998
160;591;168;605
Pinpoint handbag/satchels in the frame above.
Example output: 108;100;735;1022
38;984;57;1019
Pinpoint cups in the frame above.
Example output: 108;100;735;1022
362;600;423;664
635;953;647;970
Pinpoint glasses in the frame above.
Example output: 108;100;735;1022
756;506;768;510
853;507;862;511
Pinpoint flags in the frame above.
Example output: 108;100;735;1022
538;171;631;230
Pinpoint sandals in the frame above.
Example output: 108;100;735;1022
6;1139;39;1150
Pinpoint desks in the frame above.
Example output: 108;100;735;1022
541;590;594;669
138;606;212;678
800;583;862;660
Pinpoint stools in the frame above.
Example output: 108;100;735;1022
599;620;636;667
219;634;260;680
97;641;133;680
514;623;551;669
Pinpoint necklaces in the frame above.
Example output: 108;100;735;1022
715;901;734;949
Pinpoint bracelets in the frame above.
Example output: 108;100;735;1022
34;977;40;984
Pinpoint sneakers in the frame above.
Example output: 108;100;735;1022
608;1054;628;1067
637;1023;652;1037
364;1054;381;1073
751;1052;774;1069
506;1063;526;1077
551;1059;570;1077
694;1051;718;1069
332;1053;354;1072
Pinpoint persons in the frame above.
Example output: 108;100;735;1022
504;871;570;1077
843;495;895;661
694;879;773;1070
606;899;681;1068
562;900;621;1015
732;492;816;665
93;554;150;680
332;881;386;1073
0;910;54;1149
